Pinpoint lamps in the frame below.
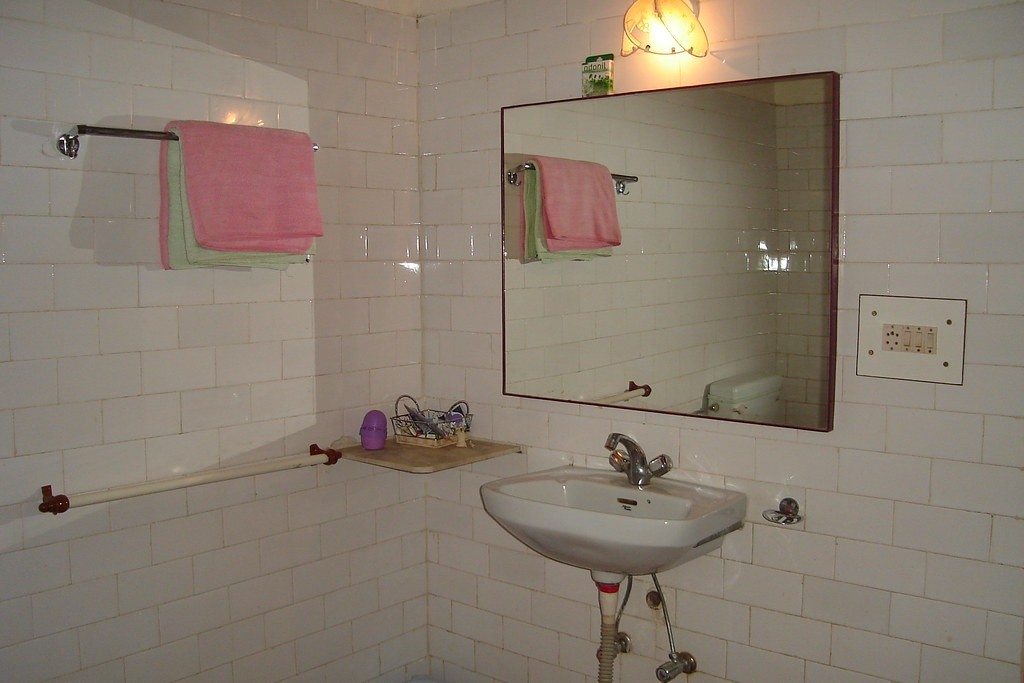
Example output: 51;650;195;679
621;0;708;57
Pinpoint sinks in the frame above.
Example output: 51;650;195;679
479;466;746;583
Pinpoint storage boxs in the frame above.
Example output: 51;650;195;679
581;54;615;96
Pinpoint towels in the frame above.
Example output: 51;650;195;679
521;158;621;264
161;120;324;270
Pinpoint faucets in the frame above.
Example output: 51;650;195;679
605;433;673;486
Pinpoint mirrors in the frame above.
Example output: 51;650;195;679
502;71;840;432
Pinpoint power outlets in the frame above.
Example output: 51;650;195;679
856;295;970;386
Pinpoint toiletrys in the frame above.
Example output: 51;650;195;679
360;411;388;449
